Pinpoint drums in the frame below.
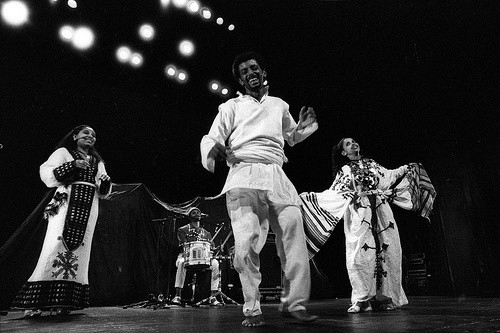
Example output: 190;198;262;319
181;241;212;272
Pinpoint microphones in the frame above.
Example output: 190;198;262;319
76;137;87;142
200;213;207;217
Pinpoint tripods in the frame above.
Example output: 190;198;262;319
122;212;241;310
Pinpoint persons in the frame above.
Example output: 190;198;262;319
298;137;437;313
172;207;220;305
199;53;319;327
10;125;112;318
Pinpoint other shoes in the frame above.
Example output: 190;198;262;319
209;296;223;306
56;308;71;315
385;302;401;311
347;301;372;312
173;295;181;304
24;308;42;317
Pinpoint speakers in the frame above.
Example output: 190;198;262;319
259;242;282;290
